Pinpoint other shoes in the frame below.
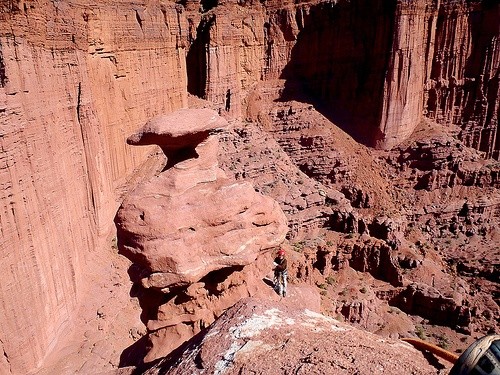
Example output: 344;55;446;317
283;291;286;297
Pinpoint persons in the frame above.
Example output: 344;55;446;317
271;250;289;296
226;89;233;112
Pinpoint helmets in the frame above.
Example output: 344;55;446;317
275;249;285;256
